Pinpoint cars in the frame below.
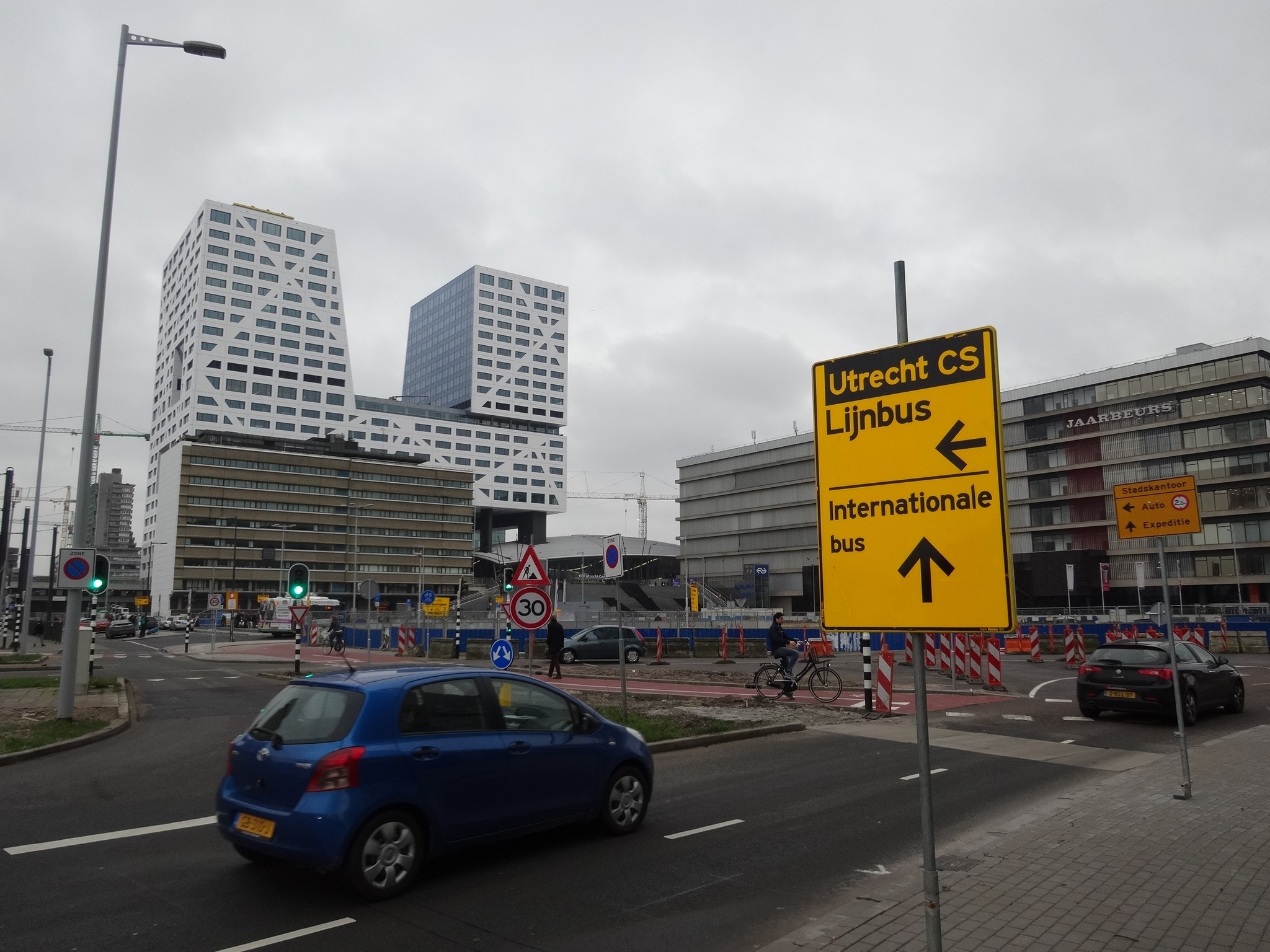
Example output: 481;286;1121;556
138;615;194;630
545;624;644;665
217;651;654;897
79;617;110;632
106;619;136;640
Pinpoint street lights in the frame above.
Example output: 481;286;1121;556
414;543;424;593
680;536;689;627
577;552;584;601
341;501;374;649
54;25;227;720
270;524;296;596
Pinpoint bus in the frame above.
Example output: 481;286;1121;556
258;594;341;636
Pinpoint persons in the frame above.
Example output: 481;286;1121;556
104;611;136;627
233;611;255;628
597;629;604;640
488;595;492;603
769;612;800;700
546;613;565;679
33;618;47;647
655;581;657;586
499;584;503;594
328;615;343;647
138;613;146;637
194;615;199;627
660;579;671;587
53;615;62;622
220;615;226;626
654;614;662;621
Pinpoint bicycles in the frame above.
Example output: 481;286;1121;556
753;640;842;703
322;632;345;655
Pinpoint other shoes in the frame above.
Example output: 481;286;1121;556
33;644;47;647
546;674;562;679
139;636;145;638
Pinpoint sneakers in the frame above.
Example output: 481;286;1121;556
783;691;795;700
784;672;796;679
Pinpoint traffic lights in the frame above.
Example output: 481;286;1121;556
85;554;110;594
288;563;310;600
503;566;515;593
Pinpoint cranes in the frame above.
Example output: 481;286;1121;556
572;472;677;538
0;414;150;549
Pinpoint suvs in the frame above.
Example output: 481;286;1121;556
1076;632;1245;726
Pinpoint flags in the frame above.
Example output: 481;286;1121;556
1136;562;1145;590
1066;564;1074;591
1178;560;1182;588
1100;563;1110;591
